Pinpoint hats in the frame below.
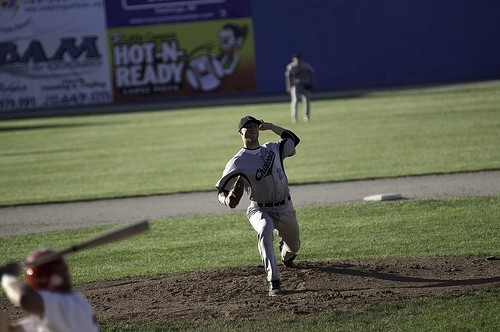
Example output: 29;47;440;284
238;115;264;133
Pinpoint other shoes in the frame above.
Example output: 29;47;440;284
278;240;297;268
269;279;283;296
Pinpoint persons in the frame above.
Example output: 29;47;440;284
0;248;99;332
215;116;300;296
286;55;315;123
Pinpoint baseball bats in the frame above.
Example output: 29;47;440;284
19;220;150;268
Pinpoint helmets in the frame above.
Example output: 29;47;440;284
24;249;72;294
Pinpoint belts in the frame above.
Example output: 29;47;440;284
257;195;291;208
